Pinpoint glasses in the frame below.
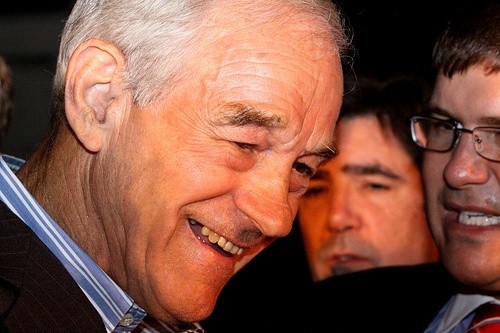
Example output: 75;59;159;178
410;114;500;163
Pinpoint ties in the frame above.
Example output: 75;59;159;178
464;302;500;333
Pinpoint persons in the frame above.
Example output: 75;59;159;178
291;65;442;285
238;0;500;333
0;0;345;333
